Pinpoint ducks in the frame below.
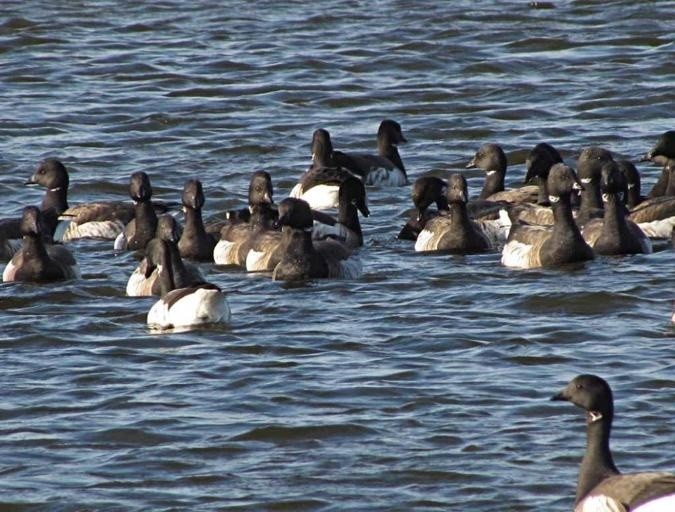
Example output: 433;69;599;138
1;120;675;333
549;373;675;512
